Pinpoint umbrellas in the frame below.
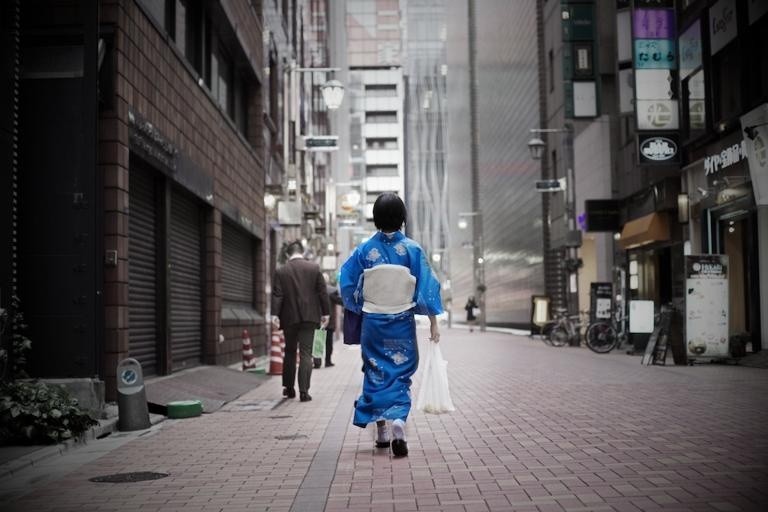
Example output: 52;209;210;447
300;392;312;401
283;388;295;398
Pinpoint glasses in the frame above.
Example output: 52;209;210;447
526;121;573;162
676;191;688;225
280;59;350;110
694;172;750;198
457;210;481;229
432;247;447;262
742;119;767;140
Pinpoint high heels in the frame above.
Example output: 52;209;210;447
266;325;302;376
240;327;258;372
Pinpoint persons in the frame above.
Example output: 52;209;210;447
270;240;331;402
313;272;344;369
465;295;478;332
341;190;444;457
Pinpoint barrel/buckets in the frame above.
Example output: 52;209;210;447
374;439;390;449
392;438;407;457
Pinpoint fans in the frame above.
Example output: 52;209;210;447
644;312;672;361
